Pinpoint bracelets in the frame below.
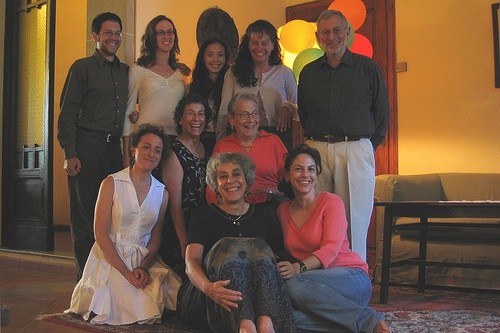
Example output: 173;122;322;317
286;101;299;109
266;187;273;199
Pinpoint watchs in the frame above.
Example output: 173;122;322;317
296;260;306;272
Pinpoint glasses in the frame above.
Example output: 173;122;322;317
231;110;260;120
96;31;125;39
155;31;175;36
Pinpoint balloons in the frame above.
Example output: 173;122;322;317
276;0;374;85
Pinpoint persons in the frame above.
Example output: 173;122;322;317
297;9;389;262
276;144;392;333
216;18;298;153
158;97;212;284
206;94;289;204
56;12;190;281
65;123;182;324
130;38;229;167
176;153;294;333
124;16;186;173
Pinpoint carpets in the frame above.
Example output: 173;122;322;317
34;285;500;333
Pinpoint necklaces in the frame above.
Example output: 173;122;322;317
228;204;249;225
157;67;173;78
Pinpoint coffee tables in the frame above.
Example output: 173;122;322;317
375;200;500;301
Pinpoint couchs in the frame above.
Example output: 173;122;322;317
373;172;500;290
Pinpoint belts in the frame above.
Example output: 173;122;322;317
306;136;369;143
88;131;121;144
